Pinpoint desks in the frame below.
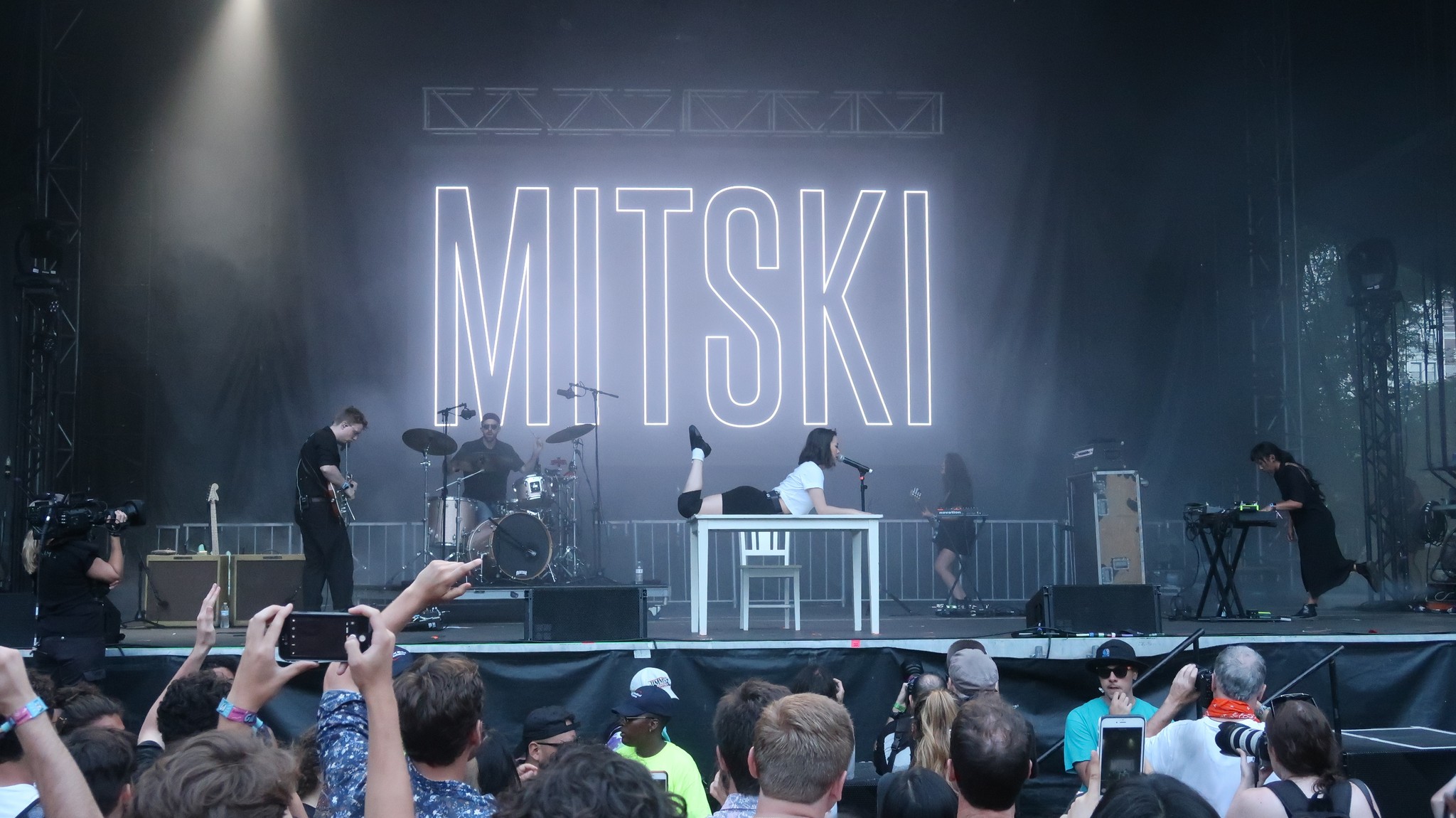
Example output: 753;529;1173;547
686;513;883;637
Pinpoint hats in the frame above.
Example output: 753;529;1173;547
482;413;500;424
629;667;680;701
513;705;581;759
611;685;672;719
1096;639;1149;669
391;645;414;679
947;640;999;696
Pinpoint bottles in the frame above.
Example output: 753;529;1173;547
635;561;643;584
220;602;229;628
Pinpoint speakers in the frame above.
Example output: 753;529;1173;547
1023;583;1161;638
142;554;229;627
233;555;305;628
522;587;649;643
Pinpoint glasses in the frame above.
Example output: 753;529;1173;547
615;713;654;723
1096;665;1128;679
1269;693;1319;719
484;424;497;430
537;736;581;748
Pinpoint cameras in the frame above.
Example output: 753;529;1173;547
1215;722;1271;763
1191;662;1216;709
903;658;925;698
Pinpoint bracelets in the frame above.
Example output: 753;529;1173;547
1256;704;1267;720
1;697;48;732
216;698;263;727
1270;502;1283;519
341;482;347;489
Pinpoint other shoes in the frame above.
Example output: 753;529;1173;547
1291;604;1318;618
689;424;712;458
1356;560;1382;592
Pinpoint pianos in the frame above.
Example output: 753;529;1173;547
1167;498;1296;623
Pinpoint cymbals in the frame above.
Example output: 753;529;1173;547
402;428;457;455
549;475;566;479
545;423;594;444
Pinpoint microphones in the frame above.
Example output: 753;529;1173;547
157;601;169;607
837;455;873;474
459;409;476;419
527;549;538;558
557;389;580;399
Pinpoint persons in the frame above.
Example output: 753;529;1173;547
1;559;1456;818
922;454;974;605
22;494;127;697
678;425;872;518
450;413;542;524
1250;441;1379;618
1376;454;1424;596
294;407;367;612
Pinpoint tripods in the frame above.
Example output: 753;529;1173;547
548;394;621;585
120;552;164;628
435;467;488;585
383;447;436;588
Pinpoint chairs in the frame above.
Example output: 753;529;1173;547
739;529;801;632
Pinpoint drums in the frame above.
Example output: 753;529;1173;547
499;497;552;527
515;474;554;507
426;490;479;550
467;513;553;581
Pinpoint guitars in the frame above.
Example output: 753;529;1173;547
910;485;940;543
198;480;231;596
326;473;357;520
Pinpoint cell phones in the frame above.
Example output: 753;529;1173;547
276;610;369;662
1098;715;1145;800
649;771;668;794
